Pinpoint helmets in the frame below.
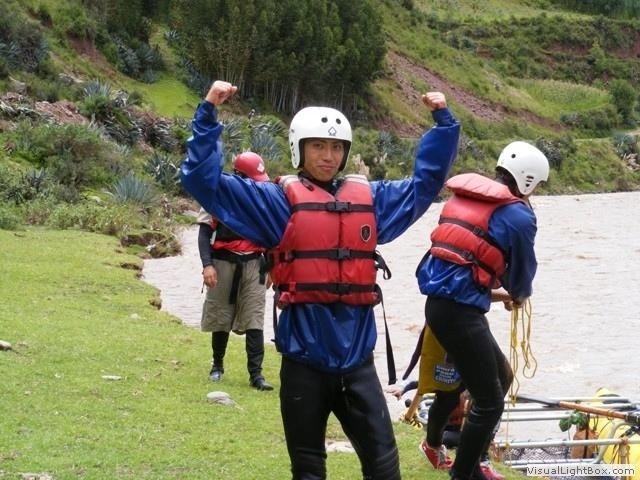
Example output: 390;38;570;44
231;151;271;183
287;105;354;173
495;140;550;197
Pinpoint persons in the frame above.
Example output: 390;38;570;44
417;137;553;480
413;287;532;480
194;150;276;392
177;78;463;480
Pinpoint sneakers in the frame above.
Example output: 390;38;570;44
418;439;456;470
480;462;507;480
250;377;274;391
209;366;224;384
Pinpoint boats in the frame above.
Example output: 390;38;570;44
399;384;640;480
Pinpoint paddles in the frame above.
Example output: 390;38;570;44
508;393;639;426
